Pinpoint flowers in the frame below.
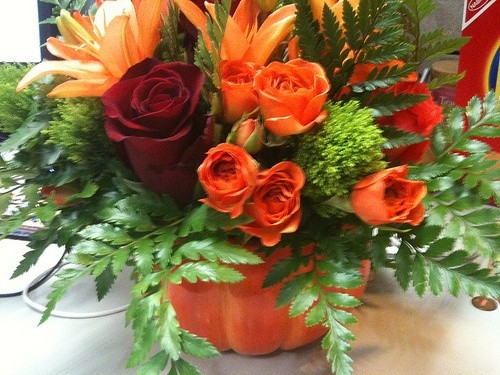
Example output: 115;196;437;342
0;0;500;374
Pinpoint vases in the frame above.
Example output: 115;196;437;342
133;222;373;359
